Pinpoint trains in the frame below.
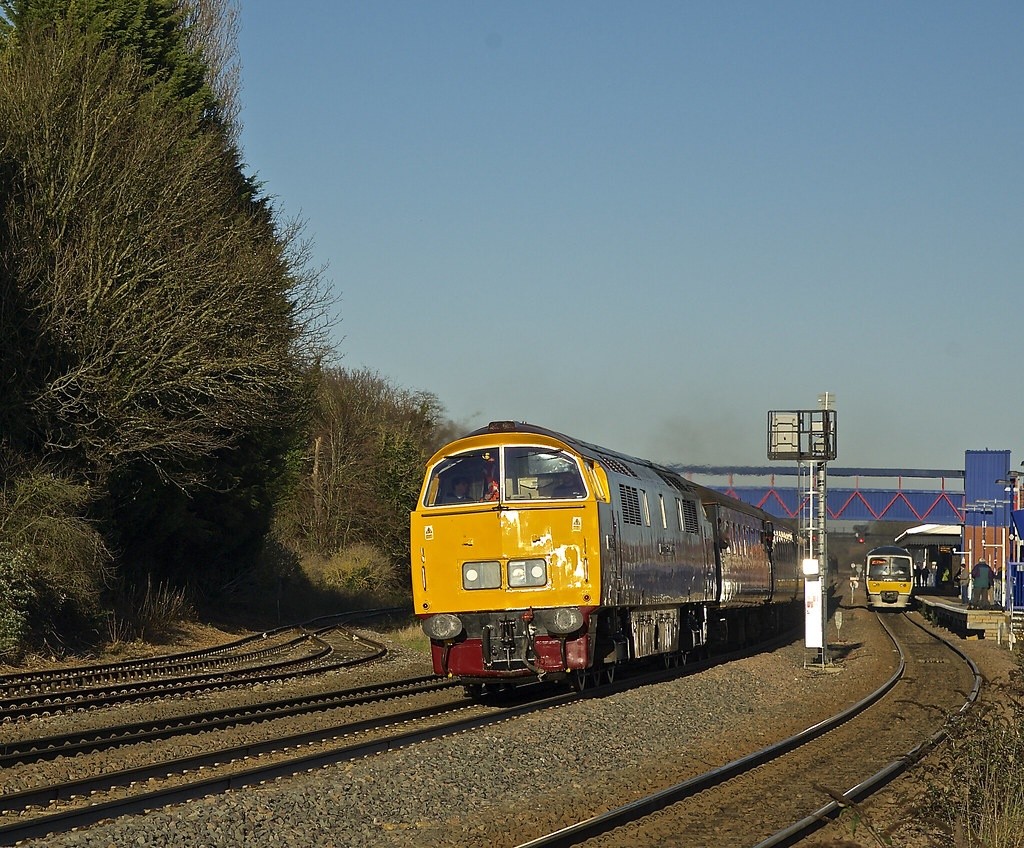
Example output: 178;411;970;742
863;545;917;613
411;419;839;702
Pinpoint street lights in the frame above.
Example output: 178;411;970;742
995;470;1024;578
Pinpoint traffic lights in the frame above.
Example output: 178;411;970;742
813;529;818;542
856;528;865;544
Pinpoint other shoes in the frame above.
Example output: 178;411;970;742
973;606;977;610
959;603;966;606
968;600;970;604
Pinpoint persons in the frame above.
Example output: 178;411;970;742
914;566;922;587
922;566;930;587
957;564;970;606
552;475;578;496
479;461;499;502
942;566;950;588
877;568;904;574
994;567;1008;580
441;477;474;502
971;557;994;609
